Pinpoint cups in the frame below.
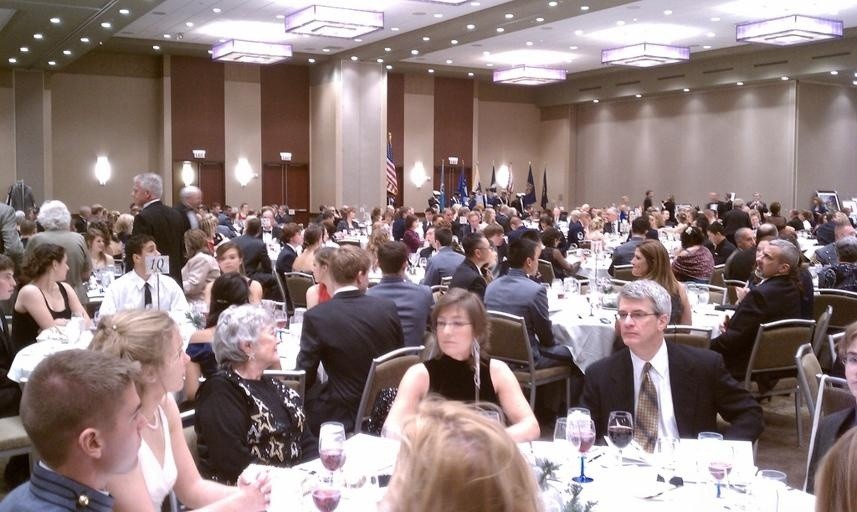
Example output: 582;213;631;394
652;436;683;470
697;431;723;472
295;307;305;335
686;280;709;310
757;469;787;511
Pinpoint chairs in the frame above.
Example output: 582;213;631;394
354;344;425;433
168;409;198;512
612;264;634;281
803;373;855;491
271;265;294;315
283;272;315;310
264;368;307;404
663;323;712;349
334;238;361;247
577;240;591;250
688;284;728;306
430;285;448;303
0;415;37;480
114;252;125;278
794;342;824;419
812;305;834;356
487;310;571;419
827;332;845;363
439;276;453;291
723;279;747;305
745;318;816;447
537;259;556;286
813;288;857;328
710;263;726;291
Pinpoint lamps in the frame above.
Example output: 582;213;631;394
492;65;566;86
285;5;385;38
601;43;690;67
211;40;294;66
410;160;427;187
409;0;474;6
495;162;510;188
180;161;195;188
95;155;112;186
235;156;254;187
736;16;843;46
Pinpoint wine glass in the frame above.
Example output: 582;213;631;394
554;417;565;469
708;441;734;500
312;475;342;512
608;410;634;471
275;310;286;342
482;411;499;423
569;415;596;485
420;256;426;271
408;252;417;275
318;421;347;484
540;276;581;307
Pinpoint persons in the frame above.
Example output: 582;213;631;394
1;173;857;512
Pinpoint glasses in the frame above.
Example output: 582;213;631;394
615;310;660;321
433;316;473;328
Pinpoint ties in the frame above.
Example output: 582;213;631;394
629;362;660;454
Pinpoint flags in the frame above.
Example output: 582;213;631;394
388;142;399;198
440;164;548;208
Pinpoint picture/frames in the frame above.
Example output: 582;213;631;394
815;189;841;211
